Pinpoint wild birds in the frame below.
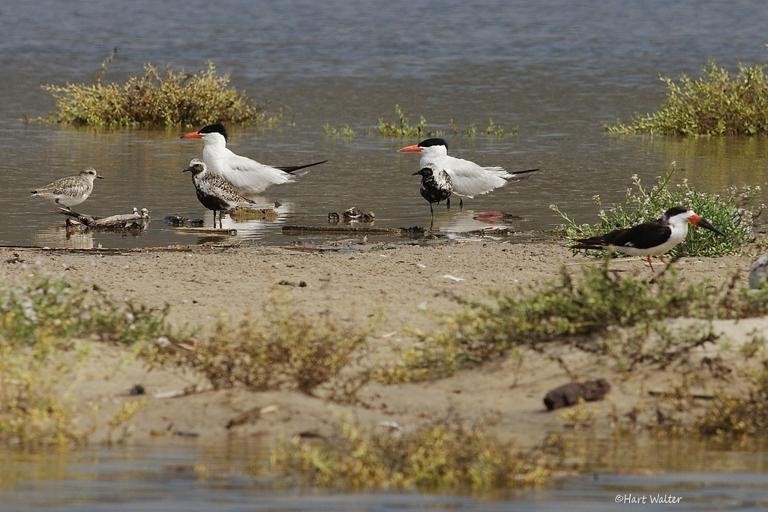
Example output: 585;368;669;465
567;206;725;273
179;124;327;200
183;157;258;229
29;166;106;213
412;166;454;228
397;138;540;211
